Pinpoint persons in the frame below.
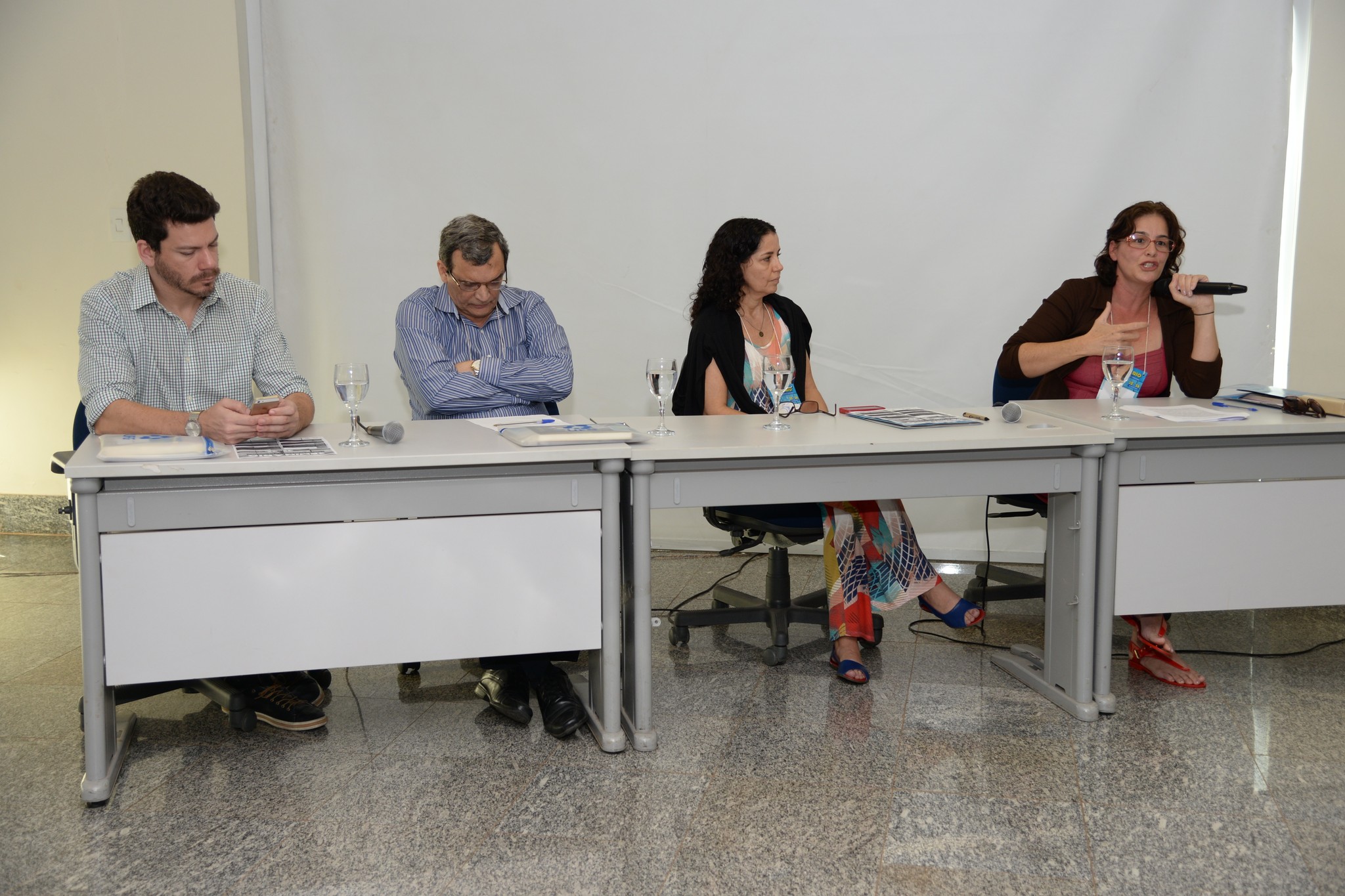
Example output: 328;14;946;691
391;208;594;740
988;197;1223;691
66;168;334;736
670;214;988;687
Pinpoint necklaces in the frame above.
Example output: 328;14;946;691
736;306;765;337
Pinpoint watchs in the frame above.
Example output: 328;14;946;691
184;408;205;437
470;359;481;378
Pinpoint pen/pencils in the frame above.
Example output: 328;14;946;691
963;412;991;421
493;419;555;427
1212;400;1258;413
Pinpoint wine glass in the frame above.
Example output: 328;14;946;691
763;354;793;431
1102;346;1134;421
646;358;678;436
335;363;373;446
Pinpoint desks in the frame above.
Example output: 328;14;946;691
61;414;628;809
575;387;1114;763
1000;371;1345;728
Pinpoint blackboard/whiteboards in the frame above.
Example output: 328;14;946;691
238;2;1308;437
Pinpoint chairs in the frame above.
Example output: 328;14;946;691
962;331;1072;627
659;492;889;668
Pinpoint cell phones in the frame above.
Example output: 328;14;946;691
249;395;279;416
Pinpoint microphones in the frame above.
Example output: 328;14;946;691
1001;402;1022;423
1177;281;1247;295
366;422;404;443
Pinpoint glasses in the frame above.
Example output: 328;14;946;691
1117;235;1176;252
779;401;837;419
446;265;507;291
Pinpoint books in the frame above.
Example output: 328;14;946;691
845;405;983;431
525;423;633;441
97;433;215;456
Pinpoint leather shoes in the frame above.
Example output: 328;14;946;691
474;669;533;725
222;671;328;731
534;666;587;739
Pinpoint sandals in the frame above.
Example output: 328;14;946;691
1121;615;1172;657
1128;640;1207;688
829;643;870;683
918;594;985;628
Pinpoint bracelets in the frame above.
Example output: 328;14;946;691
1193;311;1215;315
